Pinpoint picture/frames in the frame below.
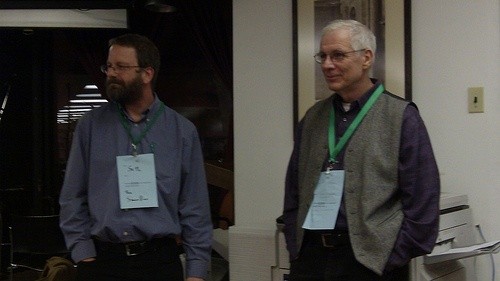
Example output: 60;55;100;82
292;0;412;148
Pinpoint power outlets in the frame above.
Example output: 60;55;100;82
468;87;484;113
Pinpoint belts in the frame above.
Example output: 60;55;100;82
93;238;173;257
303;232;350;248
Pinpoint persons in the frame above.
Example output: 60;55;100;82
59;34;212;281
283;19;440;281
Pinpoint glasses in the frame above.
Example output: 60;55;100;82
102;62;145;74
313;49;364;64
